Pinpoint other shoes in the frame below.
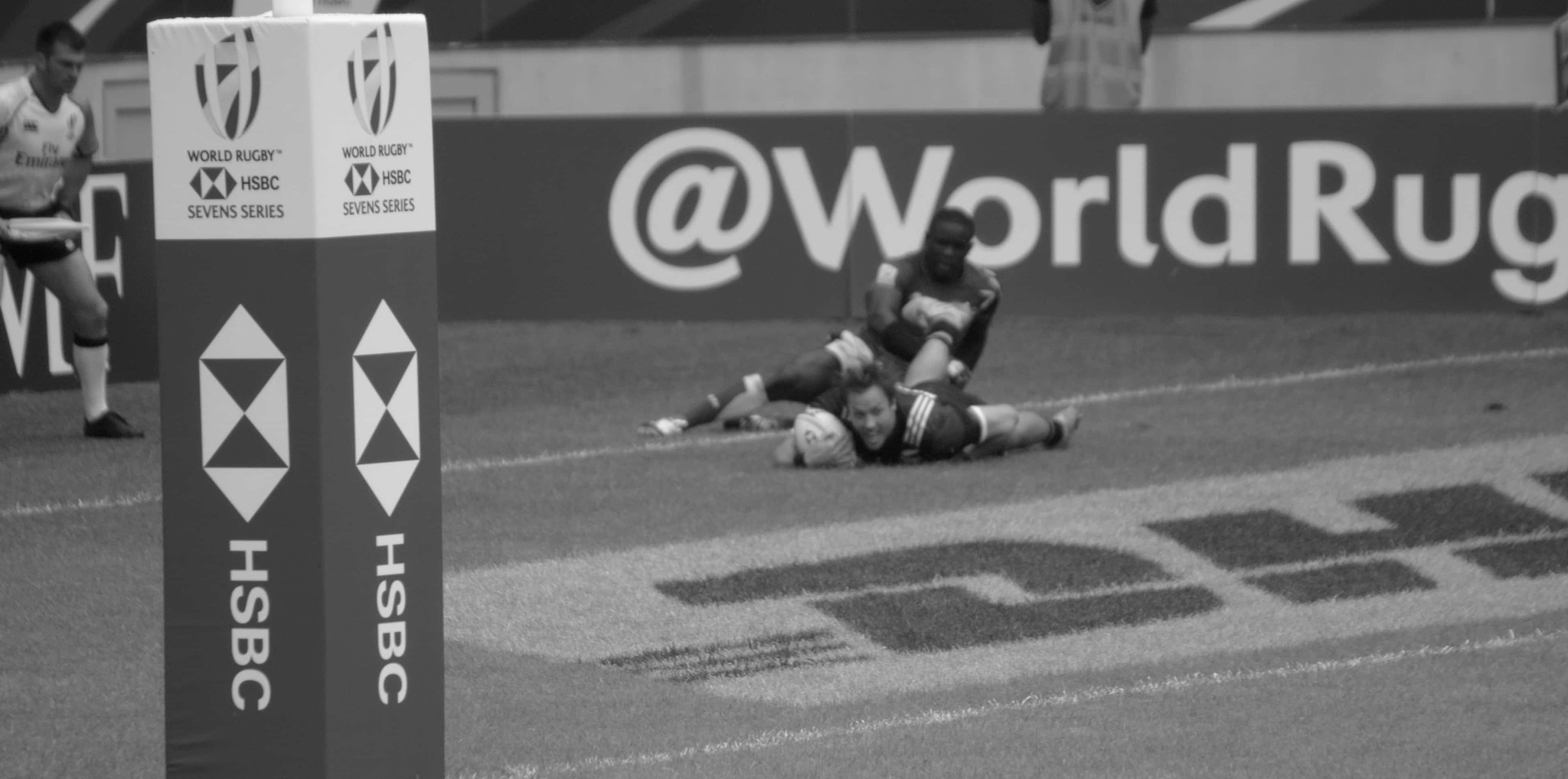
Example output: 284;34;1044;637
900;297;967;332
1042;406;1082;451
723;415;774;430
638;417;688;439
85;410;145;439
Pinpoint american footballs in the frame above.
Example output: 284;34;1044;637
792;406;862;470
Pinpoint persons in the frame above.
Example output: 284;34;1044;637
0;22;145;440
774;299;1084;467
638;207;1000;435
1033;0;1158;113
